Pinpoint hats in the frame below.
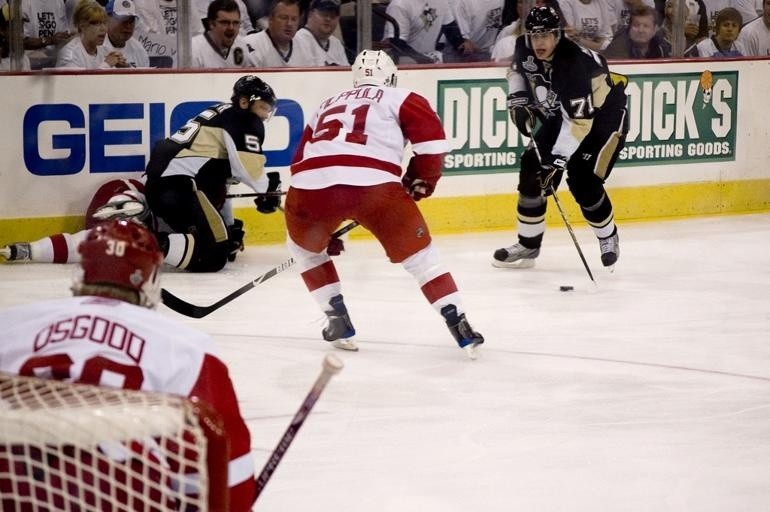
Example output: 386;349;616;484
105;0;139;20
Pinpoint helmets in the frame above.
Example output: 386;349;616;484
78;214;163;303
352;47;397;88
234;73;278;115
526;4;563;37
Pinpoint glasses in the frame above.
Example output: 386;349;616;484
214;17;244;29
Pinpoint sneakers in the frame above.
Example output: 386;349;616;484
492;241;542;262
441;301;485;348
321;295;355;341
6;239;28;261
596;226;621;268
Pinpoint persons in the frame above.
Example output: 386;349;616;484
283;45;485;351
492;5;629;268
141;73;280;273
0;175;234;269
1;1;768;71
0;216;258;512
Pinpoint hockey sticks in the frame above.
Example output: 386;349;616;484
525;120;601;295
161;222;360;319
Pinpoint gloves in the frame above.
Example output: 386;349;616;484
326;238;346;257
401;159;441;201
538;153;568;198
504;89;537;138
252;170;284;214
